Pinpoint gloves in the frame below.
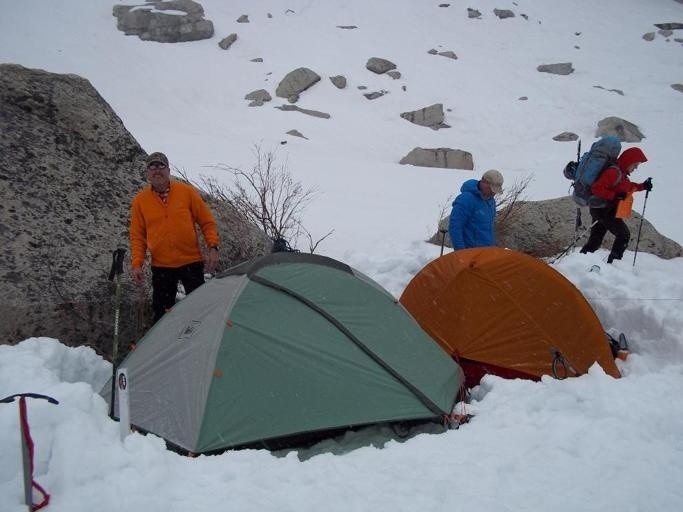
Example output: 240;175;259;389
644;179;652;191
616;192;627;200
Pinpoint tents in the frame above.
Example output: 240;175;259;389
400;248;628;379
97;252;466;458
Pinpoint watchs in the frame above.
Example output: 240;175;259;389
211;246;220;252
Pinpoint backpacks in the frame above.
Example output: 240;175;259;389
573;135;622;206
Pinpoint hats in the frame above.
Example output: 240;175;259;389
147;152;168;168
483;170;503;194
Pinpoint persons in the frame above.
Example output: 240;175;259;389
448;170;504;246
128;152;220;326
578;146;653;263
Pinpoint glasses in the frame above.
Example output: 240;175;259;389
150;164;166;169
